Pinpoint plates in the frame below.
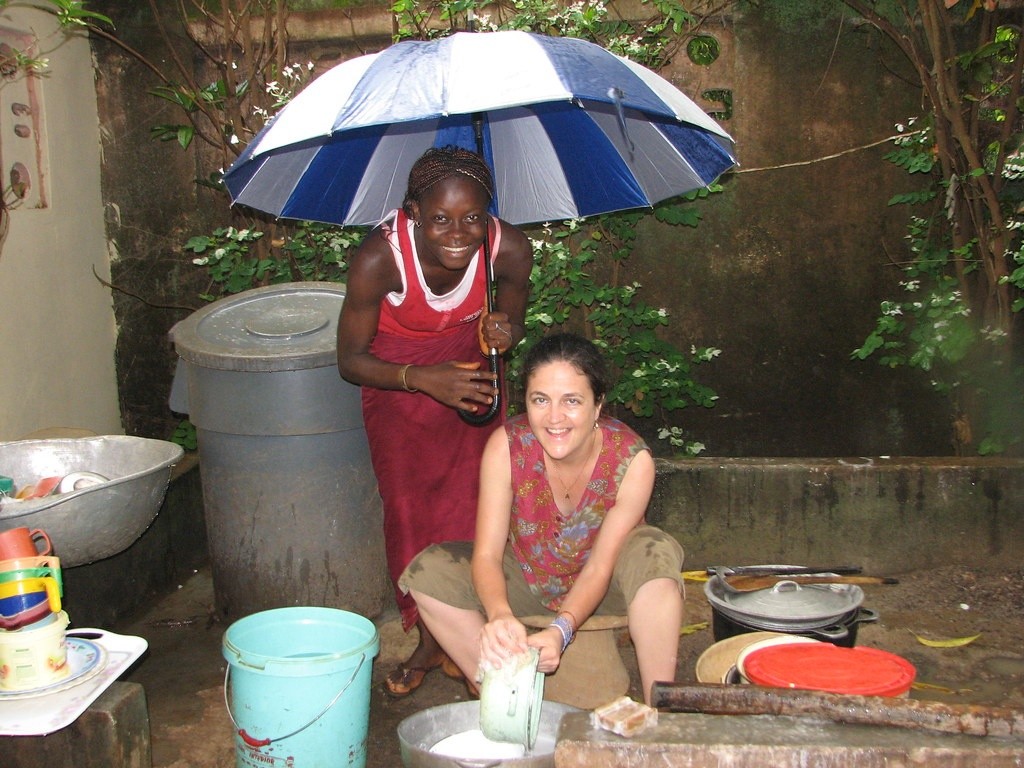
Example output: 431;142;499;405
0;642;108;700
0;637;98;694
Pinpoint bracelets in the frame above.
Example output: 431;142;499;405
399;362;417;393
549;617;572;653
480;351;490;360
556;611;577;641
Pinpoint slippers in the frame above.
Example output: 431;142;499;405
440;661;481;701
382;662;443;698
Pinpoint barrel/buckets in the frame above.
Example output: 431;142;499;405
221;606;379;768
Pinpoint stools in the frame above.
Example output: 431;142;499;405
519;614;631;710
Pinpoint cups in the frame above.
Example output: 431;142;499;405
0;526;53;561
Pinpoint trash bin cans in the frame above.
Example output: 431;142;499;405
164;277;397;631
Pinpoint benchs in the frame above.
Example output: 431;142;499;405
555;711;1024;768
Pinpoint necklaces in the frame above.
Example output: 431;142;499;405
551;426;597;500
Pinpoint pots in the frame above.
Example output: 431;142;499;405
703;565;877;647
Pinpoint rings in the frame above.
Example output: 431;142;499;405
495;322;499;330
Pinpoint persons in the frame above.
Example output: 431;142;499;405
398;331;684;708
338;145;533;697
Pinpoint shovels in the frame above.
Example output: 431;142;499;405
723;565;899;592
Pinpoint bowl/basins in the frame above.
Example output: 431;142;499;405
480;647;545;750
397;701;584;768
428;730;525;759
0;435;186;569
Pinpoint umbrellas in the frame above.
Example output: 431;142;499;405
222;31;739;430
